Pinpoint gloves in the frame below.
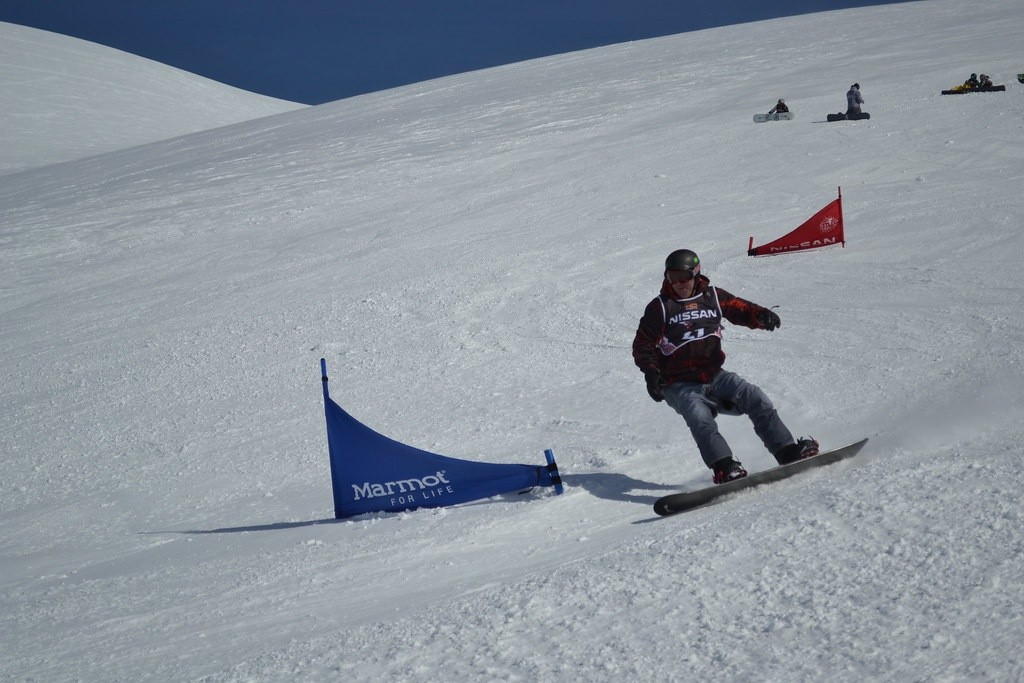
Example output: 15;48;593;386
758;306;781;331
645;367;664;402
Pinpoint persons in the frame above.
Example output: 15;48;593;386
768;99;789;114
964;73;994;90
846;83;863;115
630;248;819;485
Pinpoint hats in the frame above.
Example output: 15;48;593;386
854;83;860;89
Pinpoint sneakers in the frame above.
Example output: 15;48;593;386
774;437;819;465
713;457;747;484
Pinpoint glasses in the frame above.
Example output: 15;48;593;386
664;270;694;284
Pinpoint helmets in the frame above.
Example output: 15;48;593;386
971;73;977;80
980;74;985;80
778;99;784;104
665;249;700;272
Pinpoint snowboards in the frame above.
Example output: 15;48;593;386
941;84;1007;95
652;436;872;518
827;112;870;122
753;112;795;122
